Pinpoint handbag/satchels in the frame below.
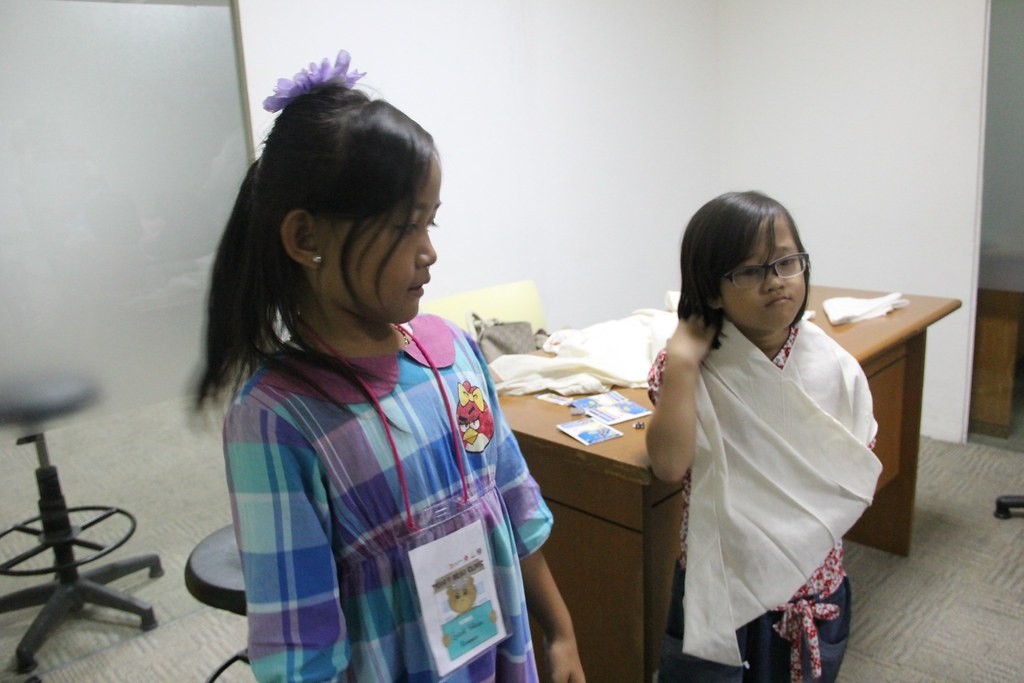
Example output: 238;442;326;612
472;312;550;365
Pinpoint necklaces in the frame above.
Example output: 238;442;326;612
396;326;408;346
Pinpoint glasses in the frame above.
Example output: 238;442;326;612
722;253;808;288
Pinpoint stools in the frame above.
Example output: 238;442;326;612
1;373;163;676
185;520;254;682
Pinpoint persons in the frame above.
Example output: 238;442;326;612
195;50;585;683
646;190;883;683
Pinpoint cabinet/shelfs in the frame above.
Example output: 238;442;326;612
970;289;1021;439
497;285;960;681
